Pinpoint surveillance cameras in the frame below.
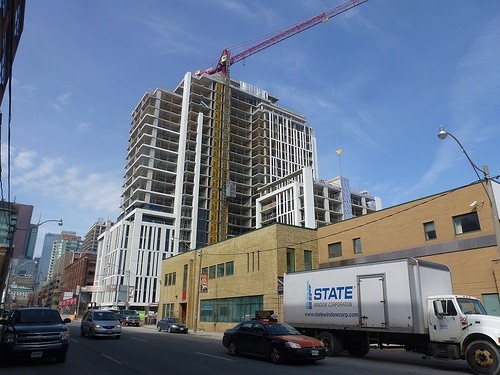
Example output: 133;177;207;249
470;201;477;207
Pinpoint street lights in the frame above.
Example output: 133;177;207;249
108;263;130;310
11;260;38;268
10;219;63;241
437;125;500;259
171;238;197;328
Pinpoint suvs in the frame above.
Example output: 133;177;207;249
0;306;72;363
119;310;140;327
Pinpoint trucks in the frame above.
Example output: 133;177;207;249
282;257;500;375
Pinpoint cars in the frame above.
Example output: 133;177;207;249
222;310;327;365
156;317;188;334
81;310;122;339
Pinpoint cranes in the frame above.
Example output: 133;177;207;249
206;3;363;74
